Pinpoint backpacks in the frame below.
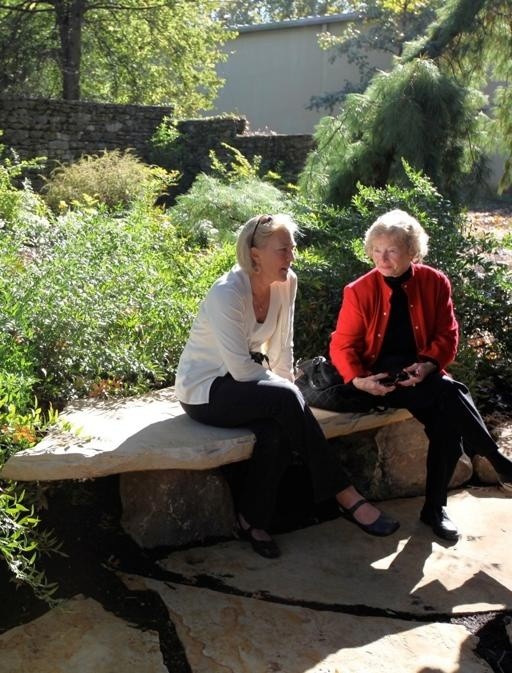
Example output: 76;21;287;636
292;355;377;414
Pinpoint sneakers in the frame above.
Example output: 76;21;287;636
418;503;460;542
497;470;512;487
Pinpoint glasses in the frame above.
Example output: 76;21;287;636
378;370;409;387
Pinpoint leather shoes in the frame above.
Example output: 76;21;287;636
234;513;282;559
338;498;401;539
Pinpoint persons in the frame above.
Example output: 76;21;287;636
330;208;512;540
174;213;401;558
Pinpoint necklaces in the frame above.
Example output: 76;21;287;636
250;282;268;312
